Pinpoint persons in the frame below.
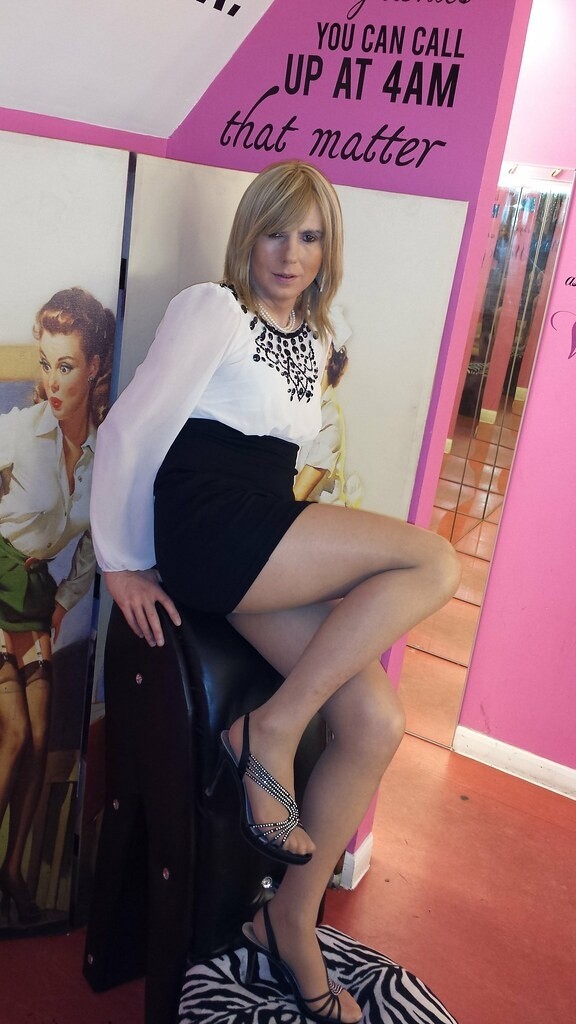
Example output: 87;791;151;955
89;164;463;1024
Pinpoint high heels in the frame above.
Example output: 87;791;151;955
205;713;312;866
242;900;361;1024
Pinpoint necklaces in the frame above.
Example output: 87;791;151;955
256;301;295;331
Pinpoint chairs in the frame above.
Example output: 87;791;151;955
122;610;459;1024
